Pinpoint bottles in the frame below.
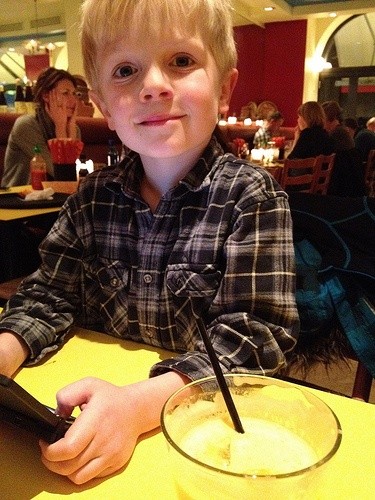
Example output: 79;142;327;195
108;140;119;166
30;144;46;190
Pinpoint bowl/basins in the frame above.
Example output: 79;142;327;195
160;372;342;499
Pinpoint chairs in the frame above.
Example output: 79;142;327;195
281;148;375;192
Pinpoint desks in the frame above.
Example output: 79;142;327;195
0;306;375;500
0;181;78;221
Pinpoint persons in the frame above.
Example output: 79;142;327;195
0;0;299;484
248;103;375;207
1;67;81;188
73;75;94;117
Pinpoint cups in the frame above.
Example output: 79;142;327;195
263;149;274;164
279;149;284;159
251;149;263;165
53;164;77;181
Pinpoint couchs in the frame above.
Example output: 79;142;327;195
0;112;297;163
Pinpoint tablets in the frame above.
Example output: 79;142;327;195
0;374;77;438
